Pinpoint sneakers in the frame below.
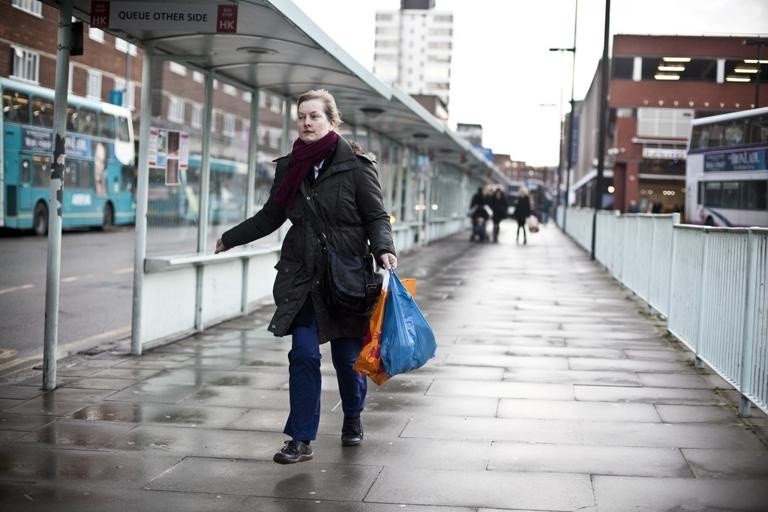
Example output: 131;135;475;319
273;440;314;465
341;417;363;446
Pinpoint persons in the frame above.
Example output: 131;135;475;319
93;140;111;194
213;88;397;466
600;194;684;225
466;185;560;246
2;93;129;143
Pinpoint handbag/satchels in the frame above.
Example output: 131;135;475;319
327;251;383;319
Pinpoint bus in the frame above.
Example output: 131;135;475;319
0;77;136;236
147;154;276;227
